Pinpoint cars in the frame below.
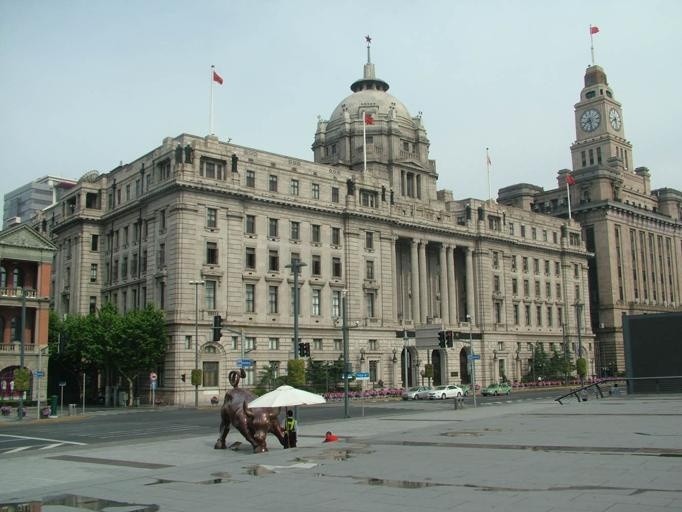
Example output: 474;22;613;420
481;383;512;396
457;385;471;396
401;386;434;400
427;385;464;399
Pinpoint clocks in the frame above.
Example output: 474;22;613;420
579;108;601;133
609;108;623;133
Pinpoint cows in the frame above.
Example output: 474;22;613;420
215;367;288;454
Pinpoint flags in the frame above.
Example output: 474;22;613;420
213;71;222;84
565;174;575;182
488;153;493;166
364;113;372;123
591;27;599;33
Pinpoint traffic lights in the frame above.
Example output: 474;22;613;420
214;315;222;341
439;330;453;348
299;343;310;357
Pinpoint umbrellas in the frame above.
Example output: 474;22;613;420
248;384;326;416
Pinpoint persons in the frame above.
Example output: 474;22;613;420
282;410;298;449
323;432;338;443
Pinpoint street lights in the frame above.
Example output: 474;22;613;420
189;280;204;408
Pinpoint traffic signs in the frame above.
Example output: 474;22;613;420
33;371;44;376
467;355;480;359
235;359;252;368
355;373;368;381
